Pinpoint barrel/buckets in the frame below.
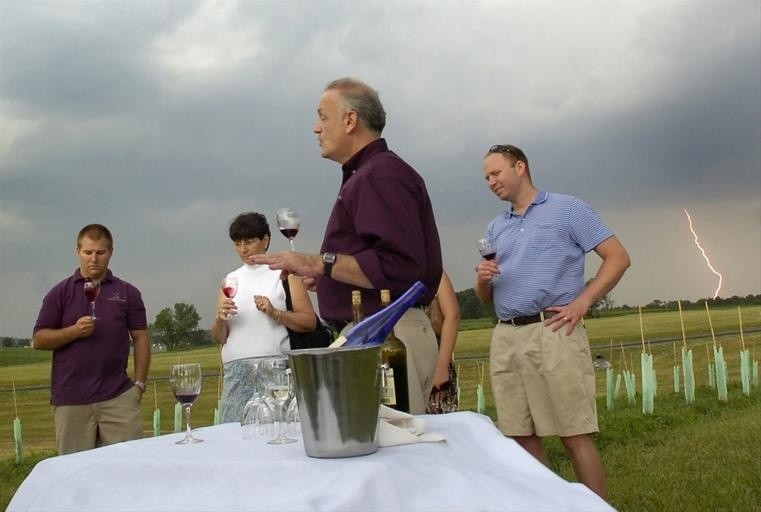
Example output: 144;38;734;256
278;331;386;460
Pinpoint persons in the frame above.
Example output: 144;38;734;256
472;142;632;504
247;75;443;416
32;223;152;456
213;211;316;424
424;266;460;417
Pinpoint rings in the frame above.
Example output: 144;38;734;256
565;317;571;322
222;311;225;314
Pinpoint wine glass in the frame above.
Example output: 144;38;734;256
220;277;240;322
476;234;506;284
168;361;205;445
240;357;298;445
83;281;103;321
275;207;303;252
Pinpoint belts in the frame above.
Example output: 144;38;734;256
501;310;560;325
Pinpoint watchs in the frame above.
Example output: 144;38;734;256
322;251;337;279
135;380;147;390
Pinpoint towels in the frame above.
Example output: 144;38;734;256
375;401;454;449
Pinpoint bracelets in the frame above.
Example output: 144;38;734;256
275;308;284;326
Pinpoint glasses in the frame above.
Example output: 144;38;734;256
489;145;518;160
431;380;453;393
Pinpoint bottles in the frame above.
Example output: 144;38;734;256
327;280;428;350
349;288;410;413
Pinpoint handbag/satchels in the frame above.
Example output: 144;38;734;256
286;303;334;349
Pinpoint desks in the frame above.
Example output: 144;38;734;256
4;410;619;512
